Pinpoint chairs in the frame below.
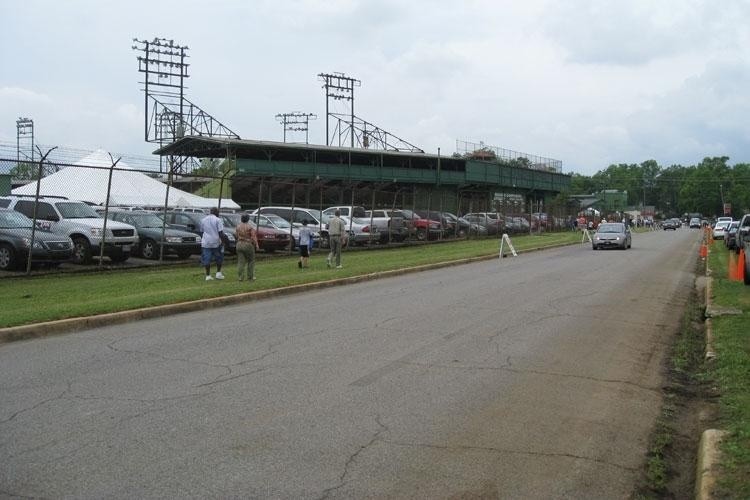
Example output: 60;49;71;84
326;258;344;270
215;273;225;280
297;258;311;268
238;276;256;283
205;275;214;281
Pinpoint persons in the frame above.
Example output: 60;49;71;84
325;209;346;269
233;213;260;282
200;207;229;281
297;218;318;269
573;215;657;230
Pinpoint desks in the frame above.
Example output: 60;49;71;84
643;173;662;216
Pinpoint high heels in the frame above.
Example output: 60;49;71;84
701;231;709;256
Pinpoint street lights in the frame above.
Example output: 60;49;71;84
643;173;662;216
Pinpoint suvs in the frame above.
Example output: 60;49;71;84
2;196;140;265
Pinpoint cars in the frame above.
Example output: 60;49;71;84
660;212;709;231
246;213;323;252
2;208;76;270
338;215;378;247
155;212;238;256
218;212;291;254
711;213;750;286
591;223;632;250
365;208;555;247
101;210;202;261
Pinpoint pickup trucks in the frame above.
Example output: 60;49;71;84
322;205;404;246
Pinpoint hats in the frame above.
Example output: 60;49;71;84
711;213;750;286
591;223;632;250
660;212;709;231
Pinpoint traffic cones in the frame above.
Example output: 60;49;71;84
701;231;709;256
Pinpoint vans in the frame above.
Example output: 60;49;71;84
251;206;331;249
